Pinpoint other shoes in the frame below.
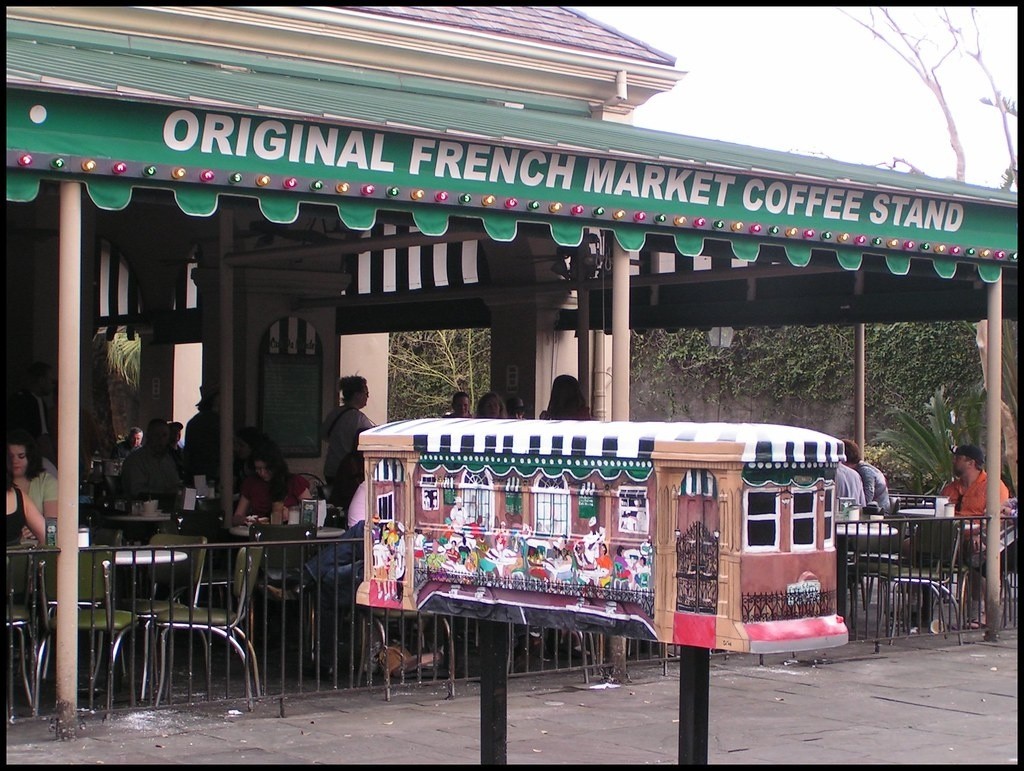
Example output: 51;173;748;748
559;641;592;658
362;656;378;673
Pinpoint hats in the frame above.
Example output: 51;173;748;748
955;445;986;467
196;381;220;407
166;420;183;430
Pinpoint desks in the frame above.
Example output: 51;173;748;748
116;549;189;566
230;526;346;538
836;524;898;617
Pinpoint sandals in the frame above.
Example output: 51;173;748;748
964;617;988;629
889;610;933;628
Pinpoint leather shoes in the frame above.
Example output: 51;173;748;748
255;569;304;606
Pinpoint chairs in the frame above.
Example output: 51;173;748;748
3;511;972;720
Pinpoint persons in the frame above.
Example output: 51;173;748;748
890;445;1018;631
515;627;590;671
320;376;373;492
6;367;111;673
476;392;505;417
259;427;364;680
443;391;472;418
109;386;312;653
539;376;591;421
835;439;890;583
507;397;525;419
